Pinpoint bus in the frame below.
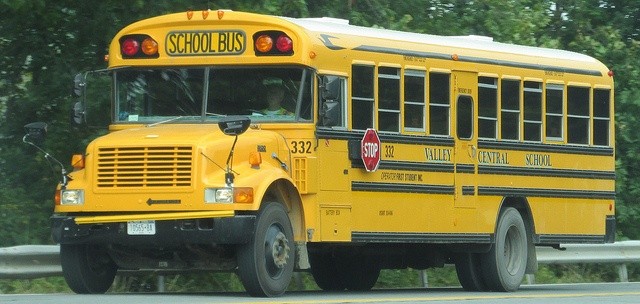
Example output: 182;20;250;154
21;7;618;298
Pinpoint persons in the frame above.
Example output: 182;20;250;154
259;87;294;117
